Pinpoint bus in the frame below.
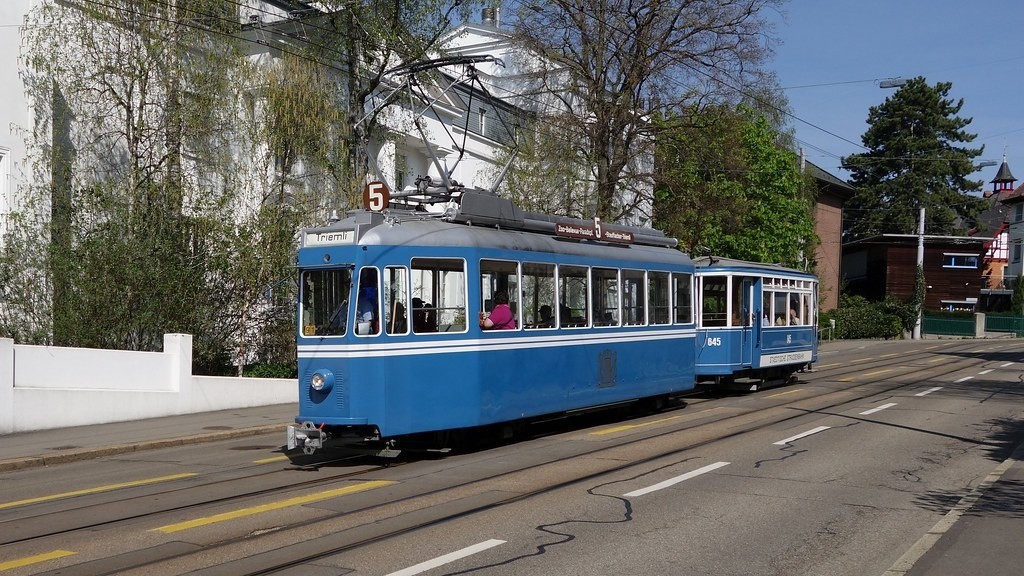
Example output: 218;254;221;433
284;53;821;453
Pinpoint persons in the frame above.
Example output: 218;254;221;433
315;279;375;335
791;308;800;324
525;303;617;329
732;300;741;326
385;302;407;334
763;307;786;326
412;298;436;333
479;289;515;330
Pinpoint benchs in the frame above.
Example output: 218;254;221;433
941;300;977;312
